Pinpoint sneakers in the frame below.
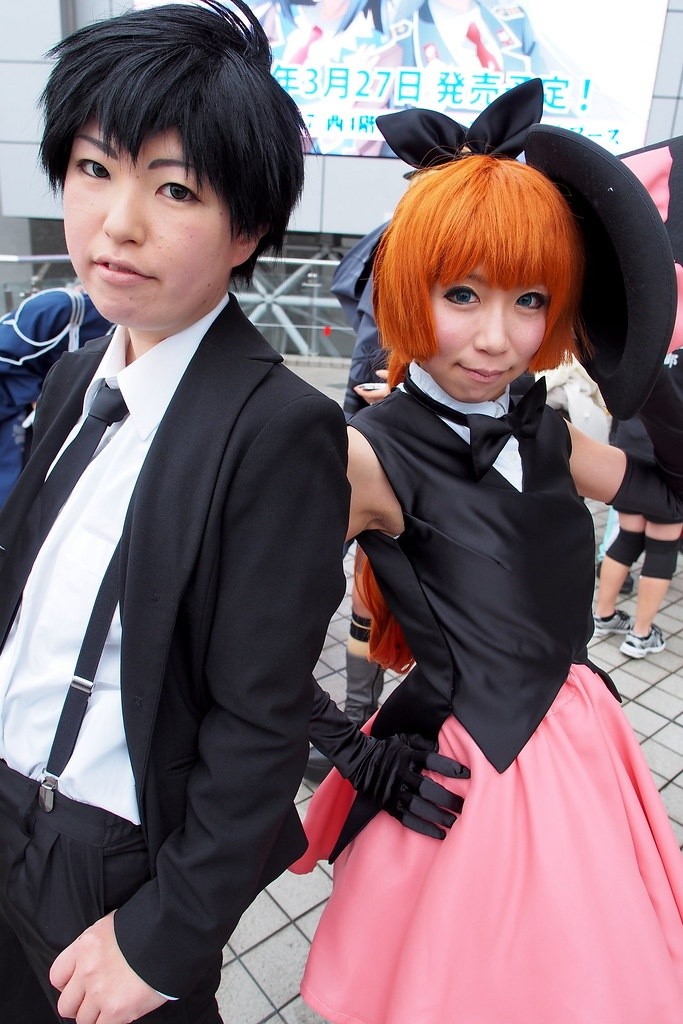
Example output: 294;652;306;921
620;623;667;660
592;609;637;637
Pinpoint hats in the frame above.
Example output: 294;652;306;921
525;122;679;422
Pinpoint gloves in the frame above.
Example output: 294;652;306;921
307;674;471;842
605;366;683;521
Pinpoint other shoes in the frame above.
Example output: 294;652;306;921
596;560;634;594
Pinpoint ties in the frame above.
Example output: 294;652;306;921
0;383;128;658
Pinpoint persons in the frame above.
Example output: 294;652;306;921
594;348;683;658
597;502;634;593
281;130;683;1024
304;218;395;783
0;288;118;506
0;0;354;1024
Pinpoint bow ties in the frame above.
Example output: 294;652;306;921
403;365;547;484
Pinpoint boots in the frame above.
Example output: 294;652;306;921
308;650;387;780
